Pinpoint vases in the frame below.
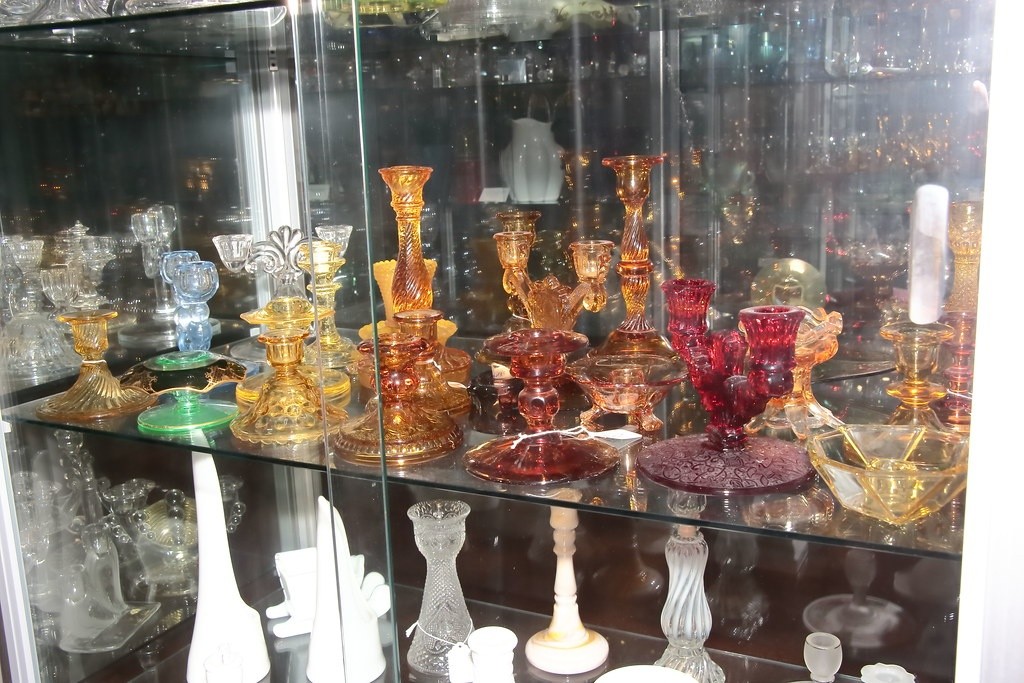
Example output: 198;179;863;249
494;112;564;203
407;500;475;676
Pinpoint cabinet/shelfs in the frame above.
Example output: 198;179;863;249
1;0;992;683
